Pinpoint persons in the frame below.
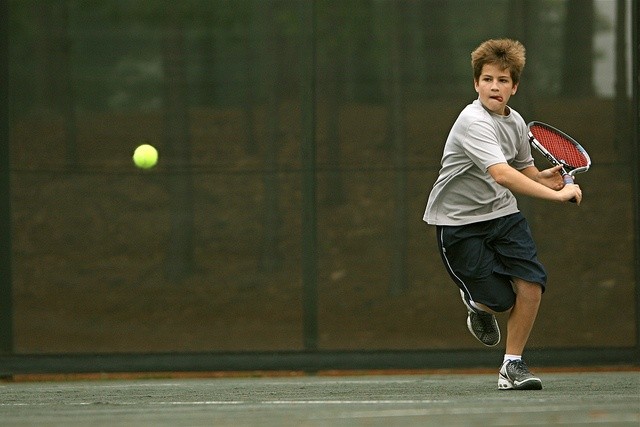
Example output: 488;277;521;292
421;38;583;391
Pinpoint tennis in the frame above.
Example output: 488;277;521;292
133;143;159;169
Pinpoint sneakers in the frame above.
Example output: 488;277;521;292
459;289;501;348
498;358;544;390
